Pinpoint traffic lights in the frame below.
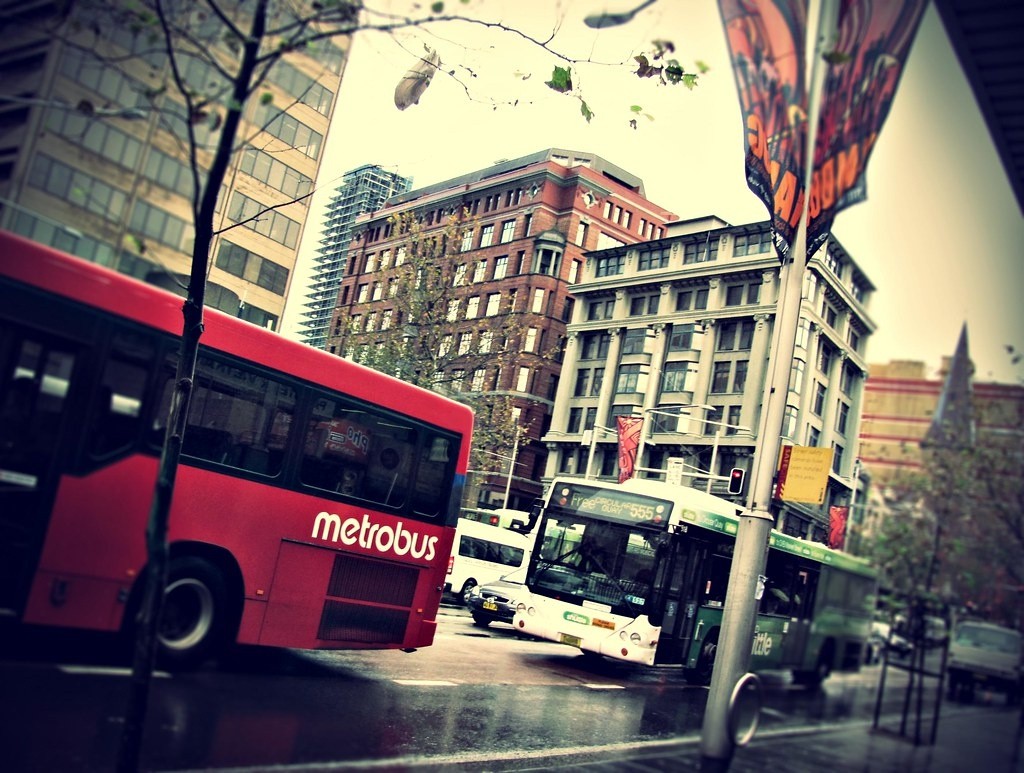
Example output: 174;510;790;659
727;467;745;495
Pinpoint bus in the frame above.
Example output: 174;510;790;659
513;476;883;698
0;226;480;692
459;508;504;530
478;505;660;599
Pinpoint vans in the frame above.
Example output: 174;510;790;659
444;517;532;605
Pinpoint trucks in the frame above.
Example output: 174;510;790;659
946;618;1022;708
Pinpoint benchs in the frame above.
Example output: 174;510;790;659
159;423;447;519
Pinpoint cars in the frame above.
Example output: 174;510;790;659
466;567;528;629
865;610;949;666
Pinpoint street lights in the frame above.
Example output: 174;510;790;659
502;425;527;508
